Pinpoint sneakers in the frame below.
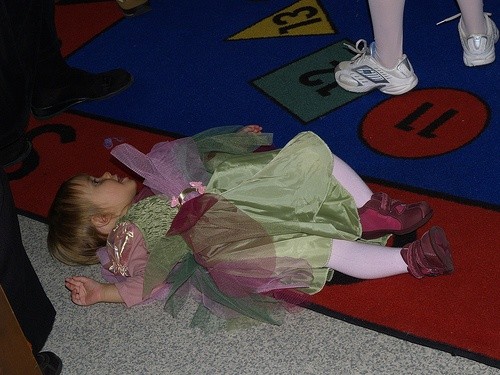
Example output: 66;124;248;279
457;12;499;67
356;191;434;240
334;39;419;95
400;227;455;279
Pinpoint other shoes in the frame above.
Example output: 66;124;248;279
36;351;63;375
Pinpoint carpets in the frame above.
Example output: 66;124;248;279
0;0;500;370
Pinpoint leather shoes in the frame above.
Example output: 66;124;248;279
31;66;133;120
0;139;33;169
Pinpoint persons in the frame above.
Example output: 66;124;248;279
334;0;500;95
47;124;454;326
0;0;153;375
